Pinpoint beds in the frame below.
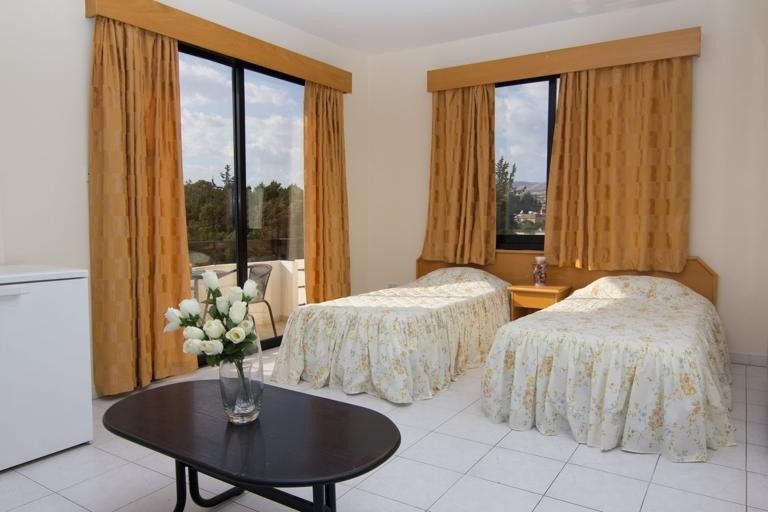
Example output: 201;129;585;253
270;250;515;405
481;251;737;462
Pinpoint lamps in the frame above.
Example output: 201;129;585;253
532;256;548;288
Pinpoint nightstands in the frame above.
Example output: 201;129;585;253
507;285;573;322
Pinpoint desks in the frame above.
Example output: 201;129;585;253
190;270;226;303
102;378;400;512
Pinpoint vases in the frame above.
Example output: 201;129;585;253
219;334;264;424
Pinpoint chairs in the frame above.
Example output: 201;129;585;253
203;264;278;338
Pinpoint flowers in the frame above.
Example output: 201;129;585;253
164;269;259;402
